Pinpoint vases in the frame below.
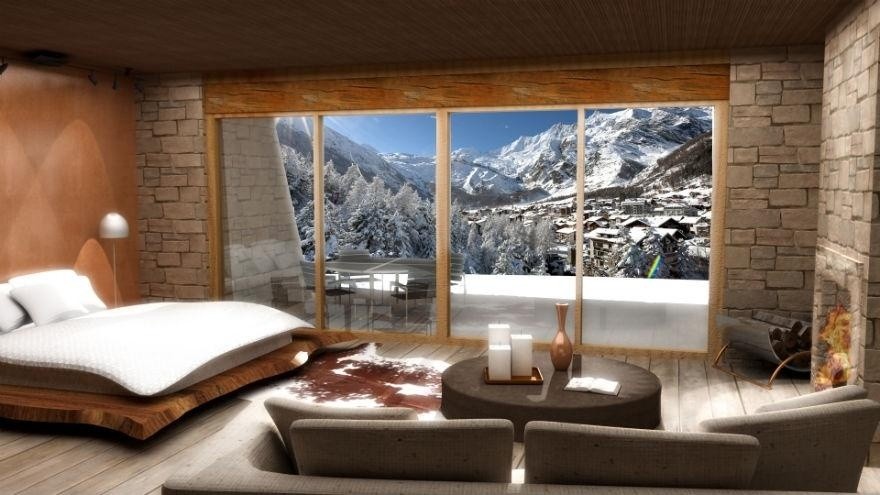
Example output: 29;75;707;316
550;302;572;372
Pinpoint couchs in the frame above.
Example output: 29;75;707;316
161;391;880;495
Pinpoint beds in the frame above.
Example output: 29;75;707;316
0;263;362;440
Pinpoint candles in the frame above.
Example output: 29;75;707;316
511;334;532;376
487;324;510;345
488;345;511;381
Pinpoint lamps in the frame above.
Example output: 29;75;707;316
98;212;129;308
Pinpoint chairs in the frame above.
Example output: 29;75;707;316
339;249;380;285
298;260;358;322
389;279;429;328
451;253;465;297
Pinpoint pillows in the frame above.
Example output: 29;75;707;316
757;381;867;412
698;396;880;493
263;394;418;455
523;421;760;489
290;418;514;483
0;282;27;334
7;270;104;328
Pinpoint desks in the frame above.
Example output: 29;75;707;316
325;260;425;305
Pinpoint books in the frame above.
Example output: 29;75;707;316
564;377;623;396
